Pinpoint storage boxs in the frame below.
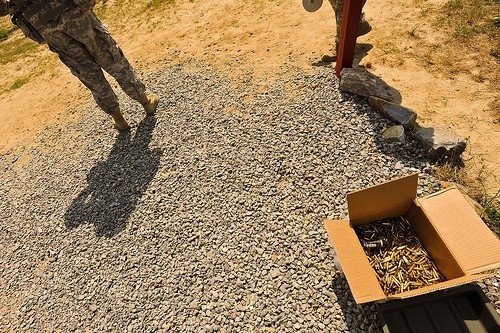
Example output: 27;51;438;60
323;174;500;309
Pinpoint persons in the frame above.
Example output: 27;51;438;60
327;0;371;55
8;0;159;130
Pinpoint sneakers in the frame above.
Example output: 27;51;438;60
335;21;372;44
110;111;130;131
139;92;159;114
321;48;339;61
